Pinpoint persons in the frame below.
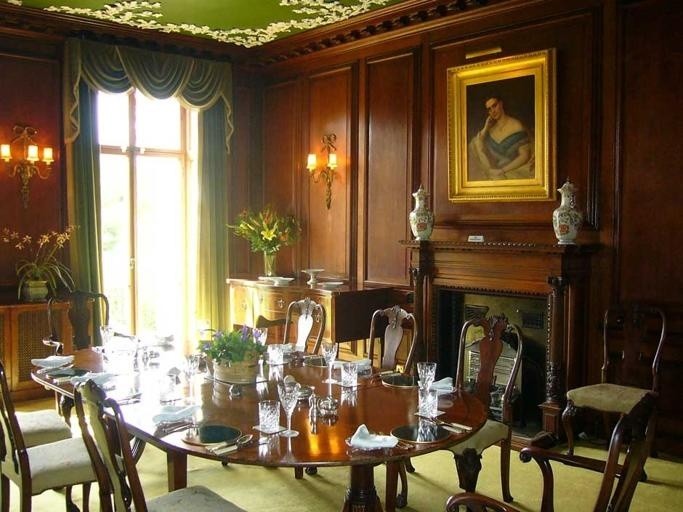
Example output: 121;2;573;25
466;93;534;182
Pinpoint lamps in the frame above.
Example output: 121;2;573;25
305;130;340;209
0;124;55;210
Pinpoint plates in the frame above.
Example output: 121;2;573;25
300;269;325;285
270;277;294;285
317;282;344;288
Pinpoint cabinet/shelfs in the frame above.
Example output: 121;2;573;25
223;274;394;345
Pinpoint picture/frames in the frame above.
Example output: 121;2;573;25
444;45;558;203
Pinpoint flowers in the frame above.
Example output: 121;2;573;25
222;200;302;268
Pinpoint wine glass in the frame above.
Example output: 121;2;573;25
37;329;473;455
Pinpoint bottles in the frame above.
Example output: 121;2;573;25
409;184;433;240
553;178;584;245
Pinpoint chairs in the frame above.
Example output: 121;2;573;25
445;391;659;512
561;297;670;482
0;290;526;512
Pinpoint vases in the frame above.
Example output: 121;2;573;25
22;280;49;302
264;253;276;276
0;221;79;301
